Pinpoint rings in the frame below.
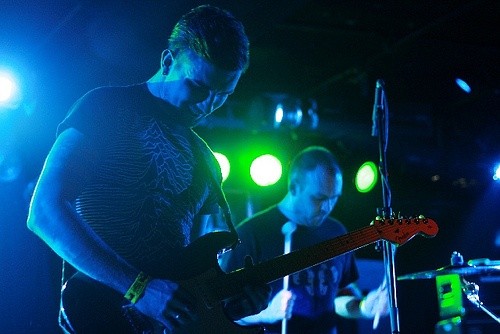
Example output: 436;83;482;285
174;312;184;320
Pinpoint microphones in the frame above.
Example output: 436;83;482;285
372;81;382;137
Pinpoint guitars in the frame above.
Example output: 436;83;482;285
61;211;439;334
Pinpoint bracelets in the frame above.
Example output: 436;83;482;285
124;272;150;304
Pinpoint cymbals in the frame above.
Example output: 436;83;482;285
397;260;500;281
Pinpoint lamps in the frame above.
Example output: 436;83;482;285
275;102;319;131
337;141;380;195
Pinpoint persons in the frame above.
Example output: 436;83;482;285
27;4;273;334
218;145;391;334
